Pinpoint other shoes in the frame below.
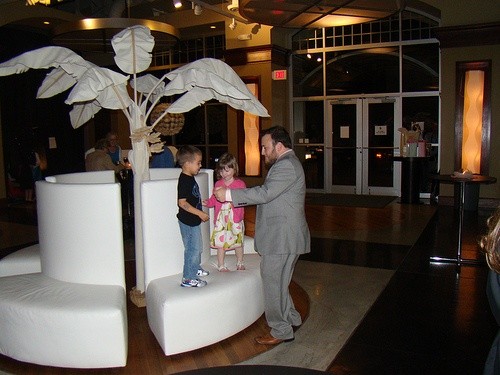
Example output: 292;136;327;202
218;265;232;273
236;263;245;270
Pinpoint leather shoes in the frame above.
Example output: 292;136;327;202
255;333;294;344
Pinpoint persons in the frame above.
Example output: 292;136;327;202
175;144;211;289
477;202;500;375
202;152;247;272
85;138;124;173
212;125;311;345
151;147;174;168
106;130;120;166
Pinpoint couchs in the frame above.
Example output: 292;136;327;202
140;171;266;356
0;170;116;276
148;168;257;256
0;181;129;368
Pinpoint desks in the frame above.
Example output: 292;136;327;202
429;173;497;274
387;155;432;206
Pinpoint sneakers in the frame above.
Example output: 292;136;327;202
180;277;207;288
196;268;209;277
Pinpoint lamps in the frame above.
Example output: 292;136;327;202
39;0;50;6
27;0;39;5
172;1;262;35
